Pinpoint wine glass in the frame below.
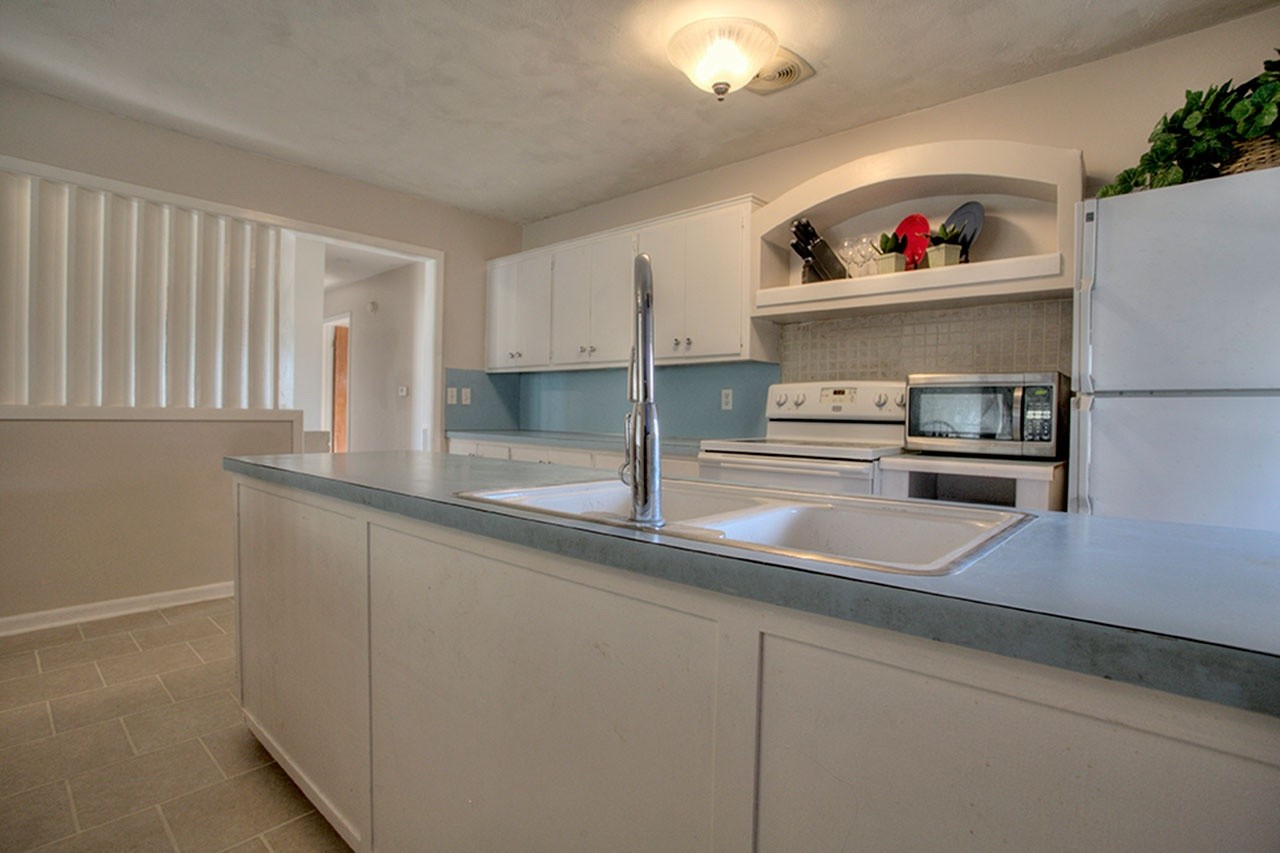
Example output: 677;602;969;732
838;235;878;279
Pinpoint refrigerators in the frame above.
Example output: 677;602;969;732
1073;165;1279;532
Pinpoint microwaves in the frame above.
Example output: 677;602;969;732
904;370;1071;457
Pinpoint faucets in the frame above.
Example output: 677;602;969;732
620;254;667;528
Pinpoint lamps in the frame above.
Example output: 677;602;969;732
666;16;779;100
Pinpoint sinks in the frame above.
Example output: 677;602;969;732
665;492;1040;579
454;473;799;539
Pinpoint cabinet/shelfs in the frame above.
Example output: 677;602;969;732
551;223;637;373
448;438;510;459
510;445;592;468
593;454;700;477
484;246;551;373
636;194;782;366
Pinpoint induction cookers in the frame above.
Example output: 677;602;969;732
699;379;909;460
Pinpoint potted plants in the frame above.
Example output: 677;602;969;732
1096;49;1280;201
922;221;966;269
869;233;909;274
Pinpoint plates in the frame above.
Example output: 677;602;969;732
944;201;985;246
894;214;930;272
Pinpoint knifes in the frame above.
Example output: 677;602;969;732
789;218;853;283
